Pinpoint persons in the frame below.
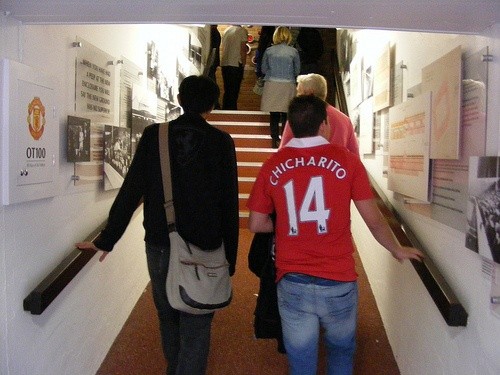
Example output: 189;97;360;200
244;91;427;375
73;74;239;375
277;72;361;162
210;25;222;108
220;24;248;109
260;26;301;148
295;25;325;73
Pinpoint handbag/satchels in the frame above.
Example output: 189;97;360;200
165;231;234;315
253;76;265;96
